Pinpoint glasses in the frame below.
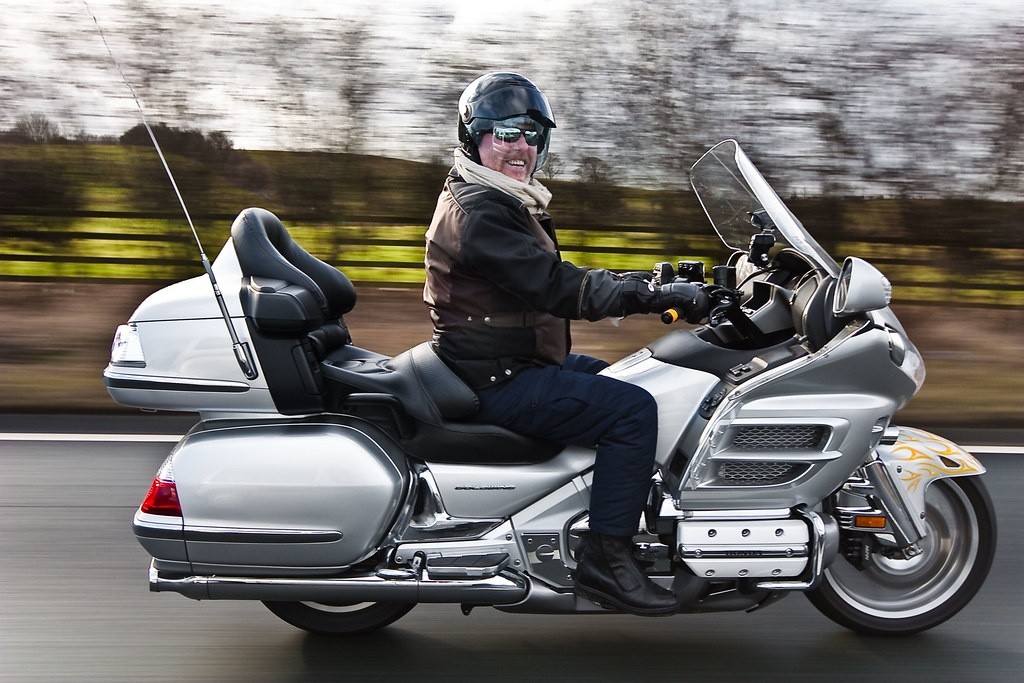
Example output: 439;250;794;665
482;124;539;146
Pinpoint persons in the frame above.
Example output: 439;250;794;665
423;70;716;618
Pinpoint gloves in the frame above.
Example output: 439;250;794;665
577;270;710;325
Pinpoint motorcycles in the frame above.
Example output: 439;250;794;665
88;16;1003;640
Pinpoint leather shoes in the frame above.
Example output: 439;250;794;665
571;536;681;618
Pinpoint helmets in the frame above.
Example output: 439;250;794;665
458;74;556;155
